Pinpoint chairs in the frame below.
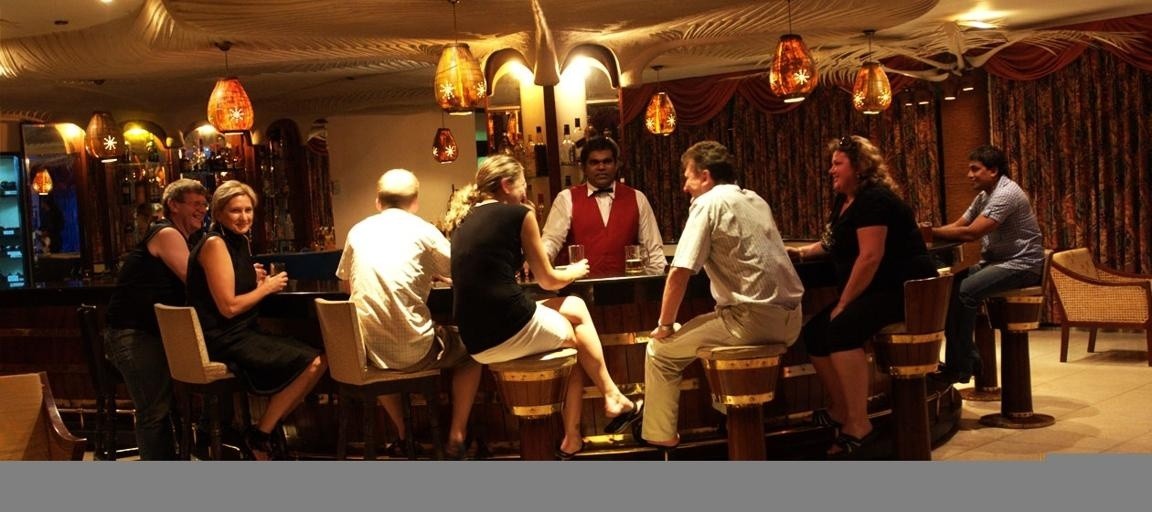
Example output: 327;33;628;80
1050;245;1152;369
0;371;88;460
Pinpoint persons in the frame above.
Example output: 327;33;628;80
530;134;670;283
785;132;938;458
178;178;328;461
922;144;1048;383
335;166;501;461
95;176;212;462
640;141;806;452
439;150;646;460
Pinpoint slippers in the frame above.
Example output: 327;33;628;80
833;433;873;457
605;402;643;434
801;410;842;426
632;422;679;448
553;440;584;460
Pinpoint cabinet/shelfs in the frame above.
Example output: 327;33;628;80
74;127;336;253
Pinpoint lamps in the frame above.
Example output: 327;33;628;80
434;0;487;118
432;105;458;164
902;79;974;107
643;64;677;138
853;28;892;115
32;145;55;197
207;38;255;136
82;78;124;168
770;0;817;103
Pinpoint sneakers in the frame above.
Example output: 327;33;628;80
247;431;281;454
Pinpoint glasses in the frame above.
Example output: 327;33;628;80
188;200;210;211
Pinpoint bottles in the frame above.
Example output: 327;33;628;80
178;137;296;255
498;126;548;178
536;193;546;237
116;132;166;255
565;175;573;189
526;184;534;203
560;117;613;165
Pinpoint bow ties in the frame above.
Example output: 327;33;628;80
593;188;613;194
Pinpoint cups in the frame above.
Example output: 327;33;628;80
288;279;299;292
920;222;933;247
568;244;584;263
625;245;651;274
270;262;284;291
